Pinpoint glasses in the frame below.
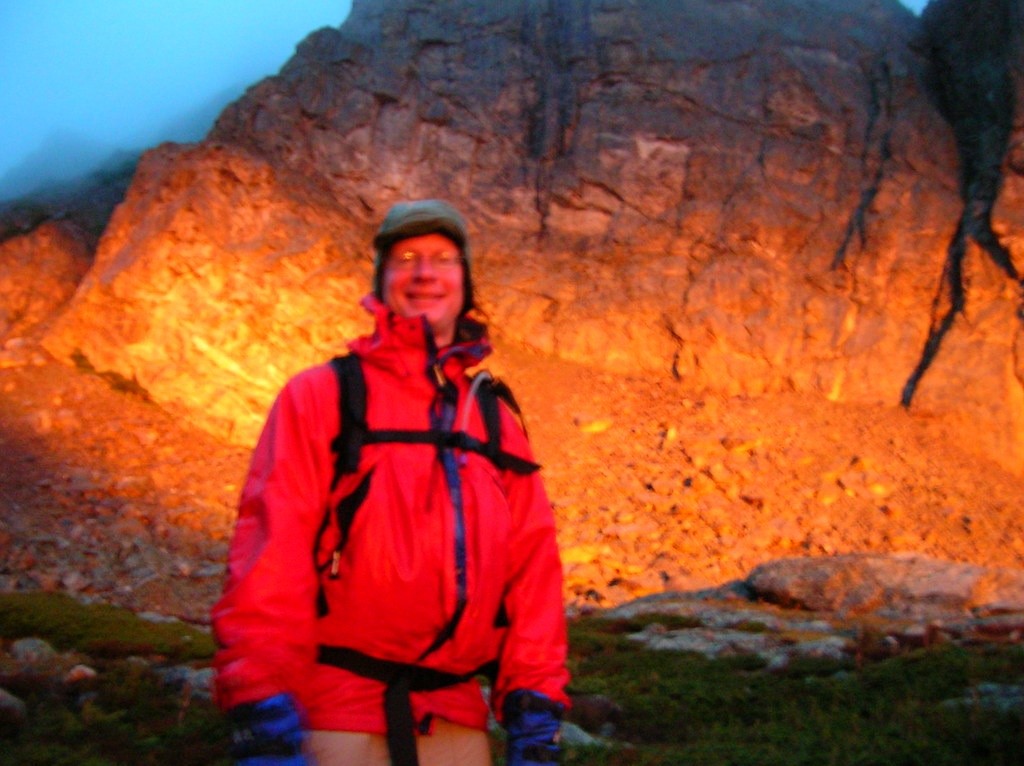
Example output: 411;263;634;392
380;251;467;269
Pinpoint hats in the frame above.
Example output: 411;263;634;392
373;200;475;307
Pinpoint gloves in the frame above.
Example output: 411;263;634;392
228;694;310;766
502;689;564;766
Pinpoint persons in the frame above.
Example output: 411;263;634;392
212;200;572;766
